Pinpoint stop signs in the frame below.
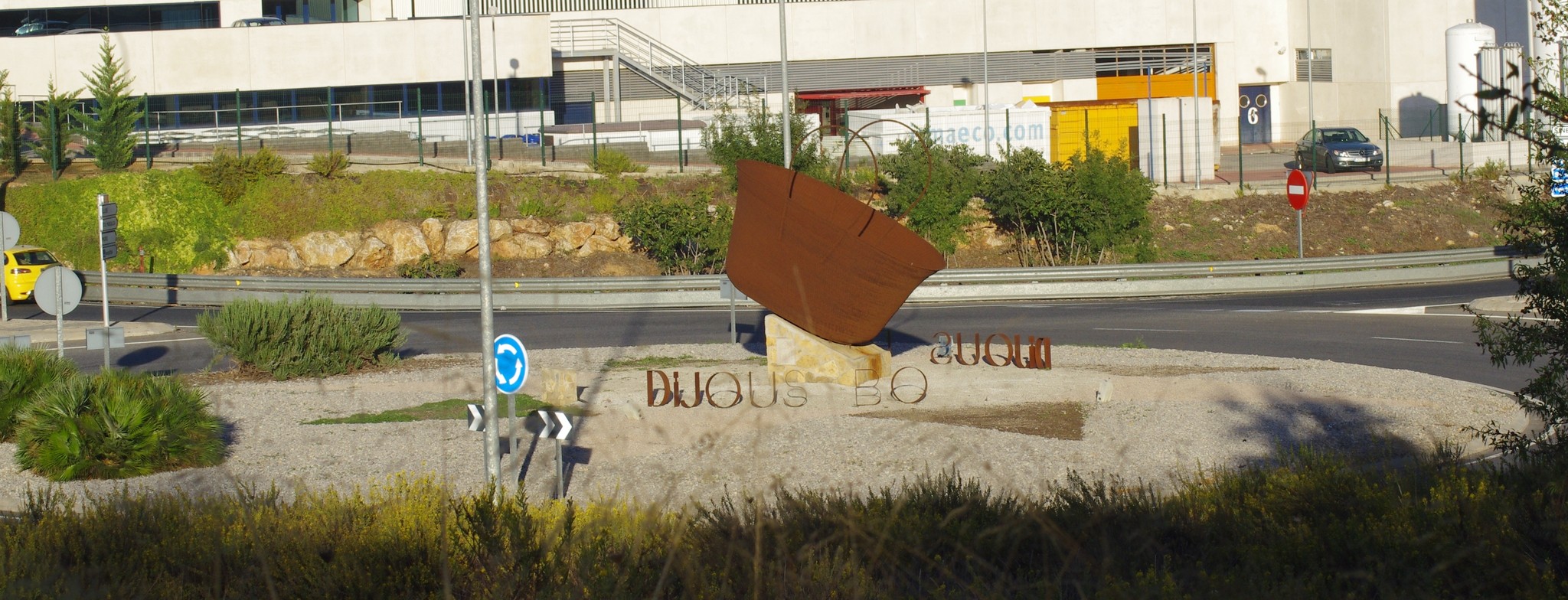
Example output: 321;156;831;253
1286;168;1307;210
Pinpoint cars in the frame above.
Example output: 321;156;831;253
1293;126;1384;174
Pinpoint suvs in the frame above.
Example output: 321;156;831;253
3;244;63;306
